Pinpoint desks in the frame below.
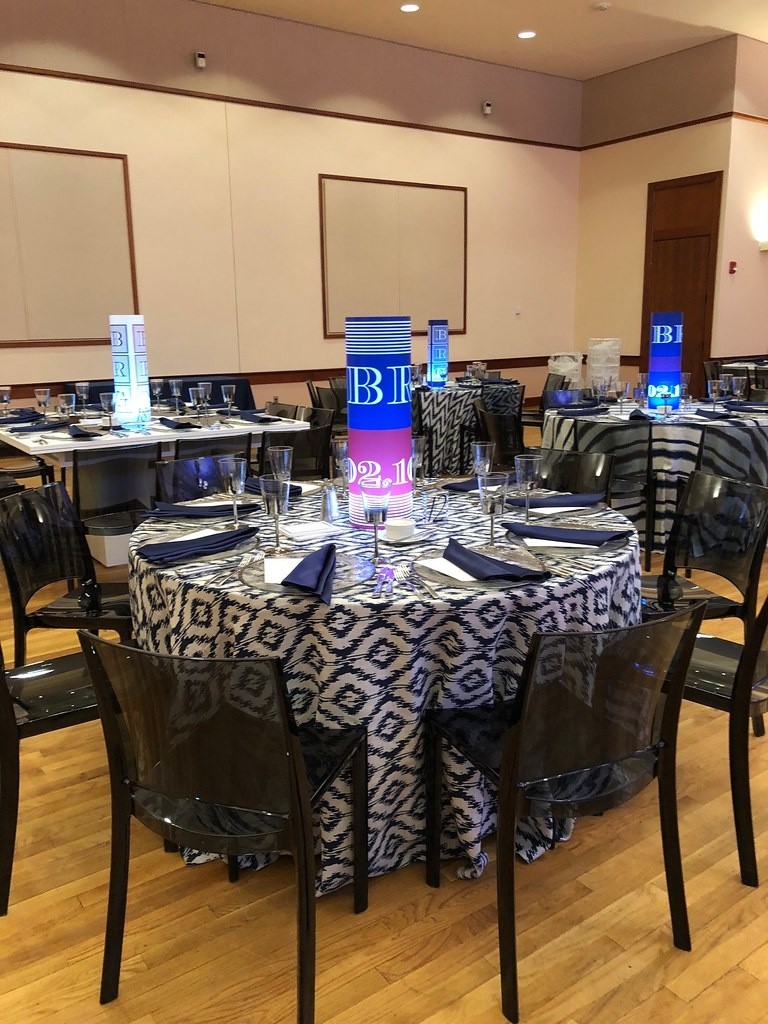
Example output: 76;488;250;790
543;399;767;554
0;410;311;528
722;362;768;387
63;376;257;419
128;475;640;895
413;380;522;473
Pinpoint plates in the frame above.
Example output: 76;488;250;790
137;529;260;563
433;477;517;496
412;547;545;589
239;551;375;595
155;499;249;524
239;480;324;499
505;493;608;517
505;522;629;555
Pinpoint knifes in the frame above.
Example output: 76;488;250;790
372;567;395;599
388;528;436;543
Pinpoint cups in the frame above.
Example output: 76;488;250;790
467;362;487;377
383;519;416;540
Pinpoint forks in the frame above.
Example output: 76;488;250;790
393;565;439;601
204;553;266;587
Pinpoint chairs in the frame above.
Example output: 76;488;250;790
0;362;768;1023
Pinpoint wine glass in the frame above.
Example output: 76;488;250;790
411;363;422;389
150;379;237;427
331;439;349;499
34;382;90;434
0;387;11;417
659;382;672;418
470;441;543;553
412;436;428;498
592;376;629;415
360;478;393;567
637;373;650;409
218;446;294;556
99;392;118;435
680;373;747;412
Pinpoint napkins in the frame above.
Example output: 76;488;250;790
440;478;516;492
9;422;68;433
68;424;103;438
139;530;261;565
695;408;740;420
159;417;203;430
140;498;260;516
506;492;607;506
241;412;281;423
188;403;229;409
503;523;634;547
725;404;767;415
0;413;45;424
282;543;337;607
557;407;609;416
218;409;265;414
630;410;653;421
243;478;303;501
698;397;735;401
443;540;550;584
